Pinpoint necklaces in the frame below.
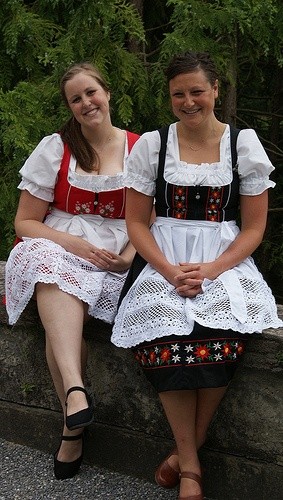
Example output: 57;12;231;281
178;120;217;152
85;128;115;157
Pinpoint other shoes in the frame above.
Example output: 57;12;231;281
64;386;95;432
155;446;183;489
176;471;204;500
53;429;86;481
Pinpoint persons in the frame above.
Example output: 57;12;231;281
109;50;283;500
0;64;155;481
